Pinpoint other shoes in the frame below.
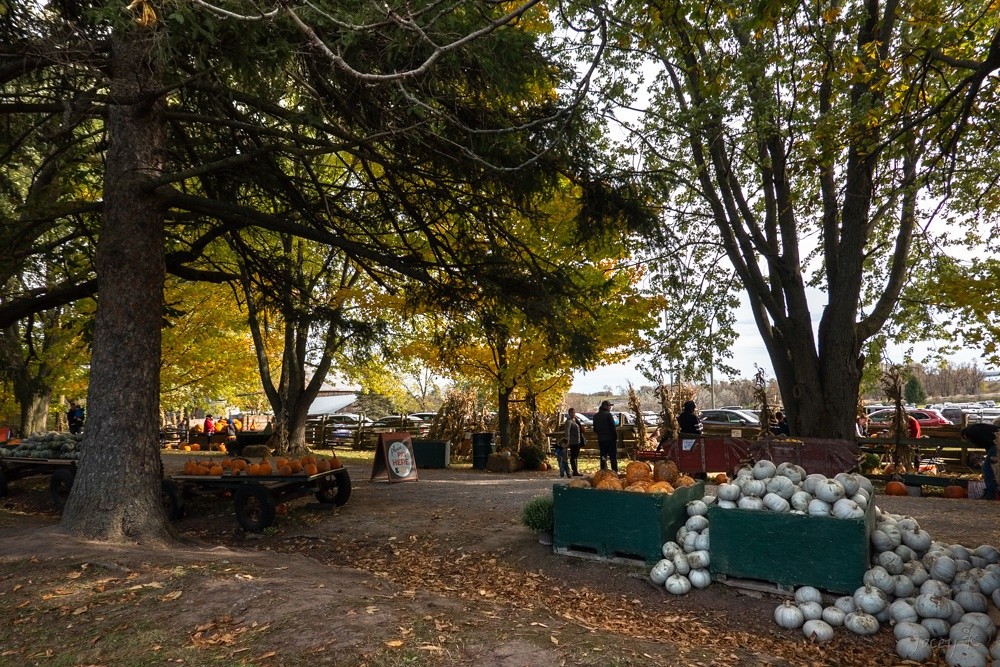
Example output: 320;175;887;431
573;471;583;476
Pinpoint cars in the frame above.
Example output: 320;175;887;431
613;412;634;424
579;412;598;420
304;418;351;444
866;408;954;428
641;411;660;425
353;416;433;447
925;400;1000;424
410;413;445;423
697;406;761;426
561;412;593;428
318;414;374;427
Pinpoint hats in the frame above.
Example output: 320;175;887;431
861;413;867;418
602;401;614;406
206;414;212;417
229;419;232;424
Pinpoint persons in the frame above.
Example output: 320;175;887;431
204;414;215;435
565;408;585;476
775;412;789;434
555;439;572;478
906;413;920;470
855;413;869;438
178;421;184;427
960;423;1000;499
68;403;84;434
678;401;701;435
226;419;239;456
593;401;621;474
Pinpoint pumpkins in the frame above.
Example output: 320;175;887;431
650;434;1000;667
502;450;511;456
568;461;696;493
179;419;242;452
184;450;341;514
0;430;84;459
539;462;547;470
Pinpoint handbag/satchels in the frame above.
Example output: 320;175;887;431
580;437;588;448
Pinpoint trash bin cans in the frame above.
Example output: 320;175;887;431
470;433;493;469
